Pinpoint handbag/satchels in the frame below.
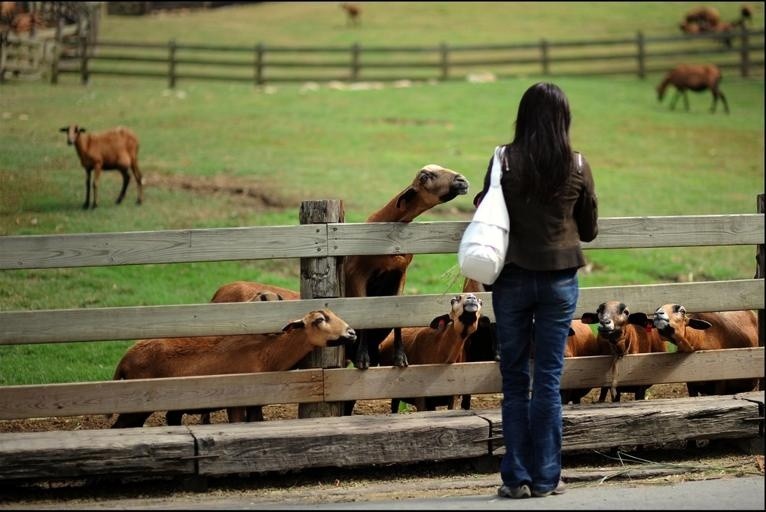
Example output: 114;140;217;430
459;171;510;285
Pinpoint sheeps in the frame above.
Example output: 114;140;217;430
580;300;669;403
646;302;761;397
55;122;146;209
654;62;730;115
104;281;594;427
341;163;471;417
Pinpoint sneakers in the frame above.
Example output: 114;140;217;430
498;481;568;498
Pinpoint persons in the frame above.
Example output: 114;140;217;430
474;82;598;499
